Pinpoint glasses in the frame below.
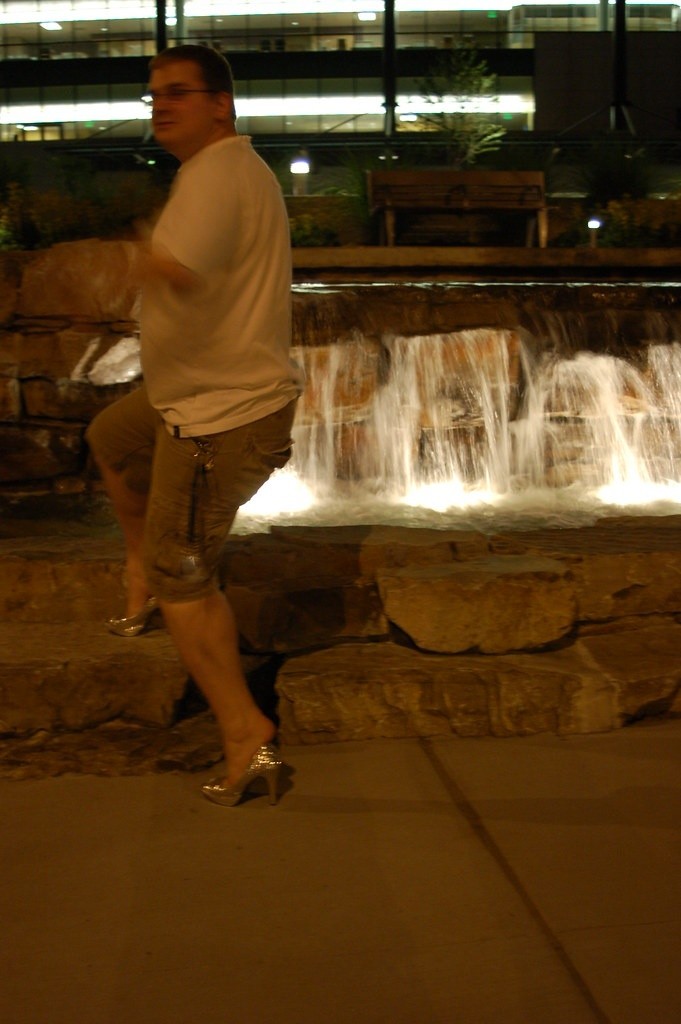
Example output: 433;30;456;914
140;86;209;105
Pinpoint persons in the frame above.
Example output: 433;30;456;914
85;45;304;807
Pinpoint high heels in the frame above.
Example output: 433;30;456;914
106;596;158;636
200;742;283;807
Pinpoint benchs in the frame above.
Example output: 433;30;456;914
365;168;552;248
